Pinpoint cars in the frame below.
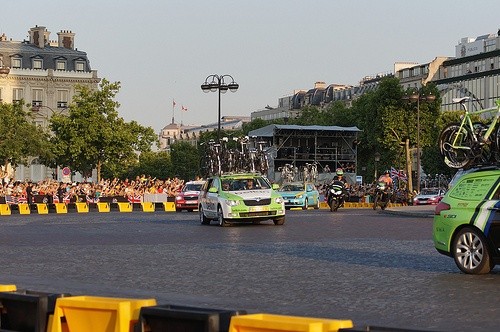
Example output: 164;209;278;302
198;172;286;227
413;187;446;205
275;183;320;210
432;169;500;275
174;181;207;212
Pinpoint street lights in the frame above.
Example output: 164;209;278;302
32;105;68;180
402;87;436;193
200;74;239;129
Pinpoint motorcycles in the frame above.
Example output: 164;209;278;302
373;176;392;211
326;180;347;212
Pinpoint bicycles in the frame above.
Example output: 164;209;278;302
277;162;318;183
438;96;500;169
199;135;269;177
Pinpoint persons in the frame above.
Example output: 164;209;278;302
0;163;257;202
318;168;420;207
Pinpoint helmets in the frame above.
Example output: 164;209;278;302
384;170;391;174
336;170;344;175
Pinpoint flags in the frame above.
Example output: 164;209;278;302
183;106;187;111
391;167;408;182
